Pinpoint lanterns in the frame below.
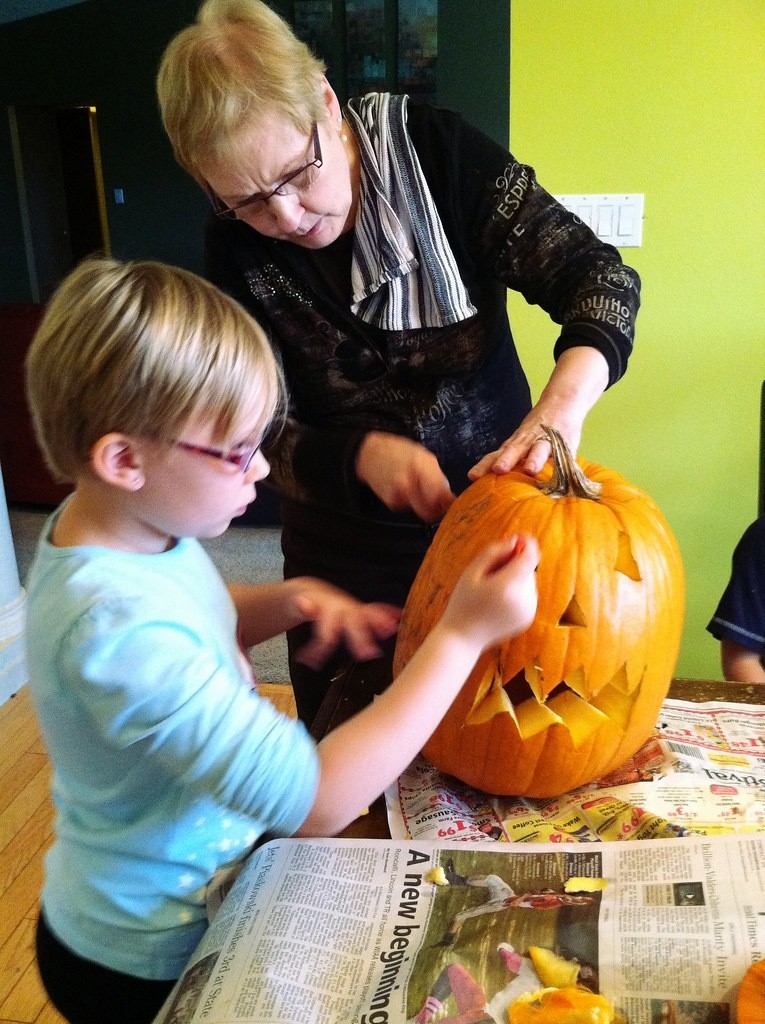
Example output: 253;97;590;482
391;425;685;797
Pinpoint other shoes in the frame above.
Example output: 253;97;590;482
429;940;456;947
445;859;457;886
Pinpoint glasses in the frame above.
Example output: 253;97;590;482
138;435;265;473
203;110;323;220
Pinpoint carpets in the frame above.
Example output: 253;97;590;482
8;510;290;685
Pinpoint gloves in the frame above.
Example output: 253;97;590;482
502;896;518;907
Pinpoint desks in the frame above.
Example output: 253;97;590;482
307;661;765;838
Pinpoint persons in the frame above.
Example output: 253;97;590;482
429;858;593;947
706;516;765;684
156;0;640;722
407;943;542;1024
25;261;539;1024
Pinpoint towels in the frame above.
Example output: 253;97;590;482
341;89;478;332
